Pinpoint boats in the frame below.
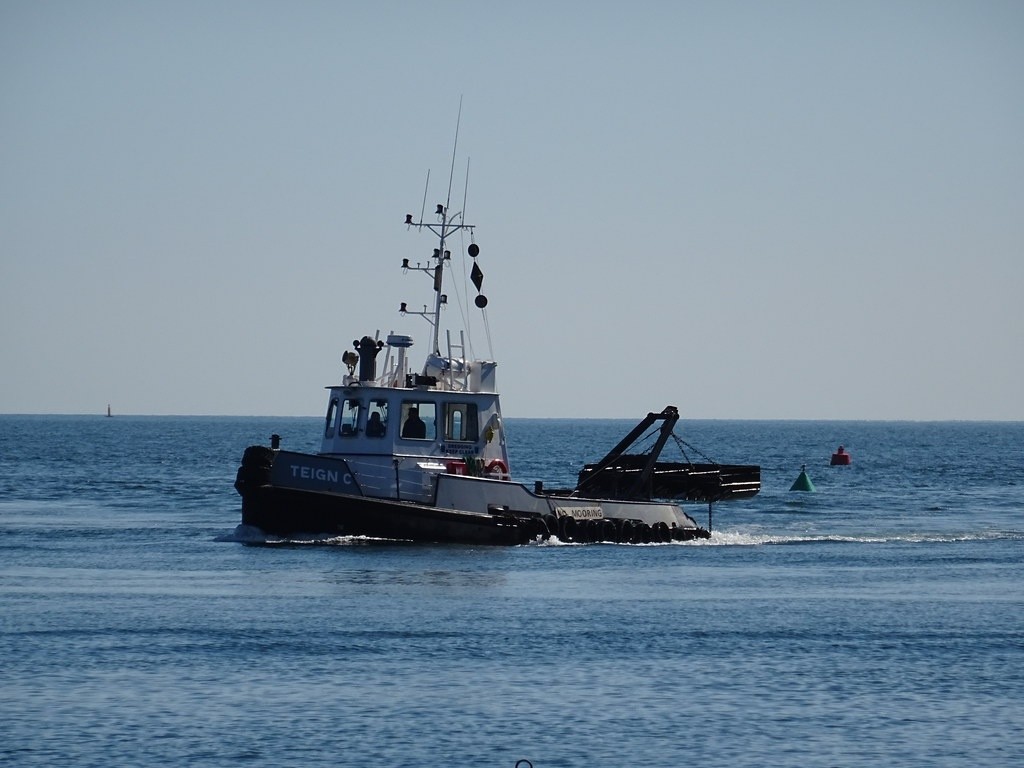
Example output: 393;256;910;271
233;88;713;547
576;405;761;503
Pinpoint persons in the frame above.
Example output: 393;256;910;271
402;408;426;439
366;412;386;438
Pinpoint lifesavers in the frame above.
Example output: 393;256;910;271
618;518;634;545
582;518;599;543
635;522;651;544
543;512;560;538
486;459;507;481
600;518;617;544
651;520;672;545
560;514;578;542
